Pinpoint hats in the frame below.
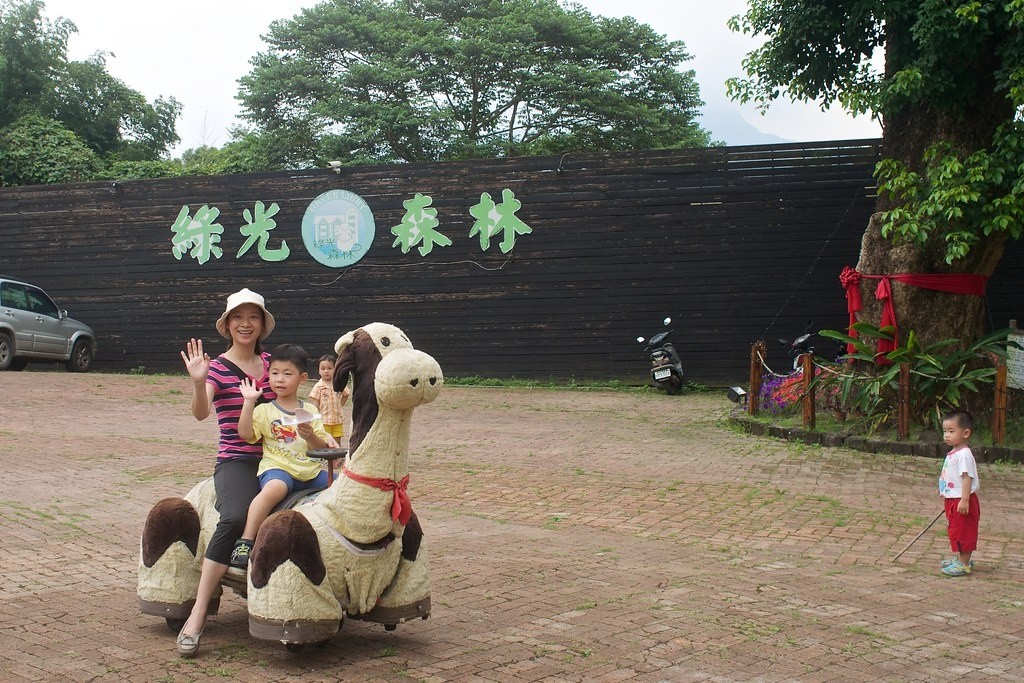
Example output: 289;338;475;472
216;288;275;342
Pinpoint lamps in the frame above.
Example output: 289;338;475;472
727;387;747;403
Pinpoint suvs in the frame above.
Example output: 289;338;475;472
0;275;99;373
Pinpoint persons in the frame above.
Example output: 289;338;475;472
939;411;981;577
177;288;342;656
307;354;351;468
229;343;339;569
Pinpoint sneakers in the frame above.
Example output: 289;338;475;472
230;539;254;567
941;556;971;568
940;561;971;575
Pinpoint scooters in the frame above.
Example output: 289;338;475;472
779;317;820;374
635;315;685;394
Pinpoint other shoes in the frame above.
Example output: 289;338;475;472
177;618;205;655
